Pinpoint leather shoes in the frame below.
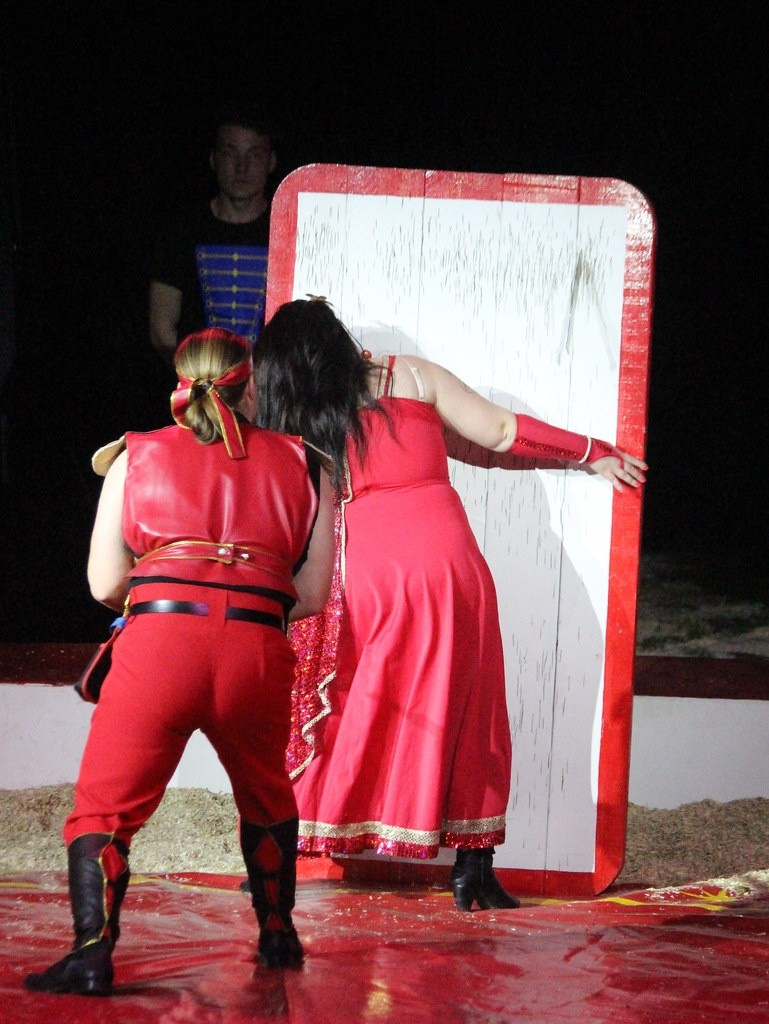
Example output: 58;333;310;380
23;946;114;995
256;930;303;967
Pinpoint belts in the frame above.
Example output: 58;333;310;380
126;600;287;631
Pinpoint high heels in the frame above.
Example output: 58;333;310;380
450;849;520;911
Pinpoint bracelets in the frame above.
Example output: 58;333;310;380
577;434;592;464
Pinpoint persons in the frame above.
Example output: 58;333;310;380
22;328;336;996
150;105;278;372
250;299;648;912
